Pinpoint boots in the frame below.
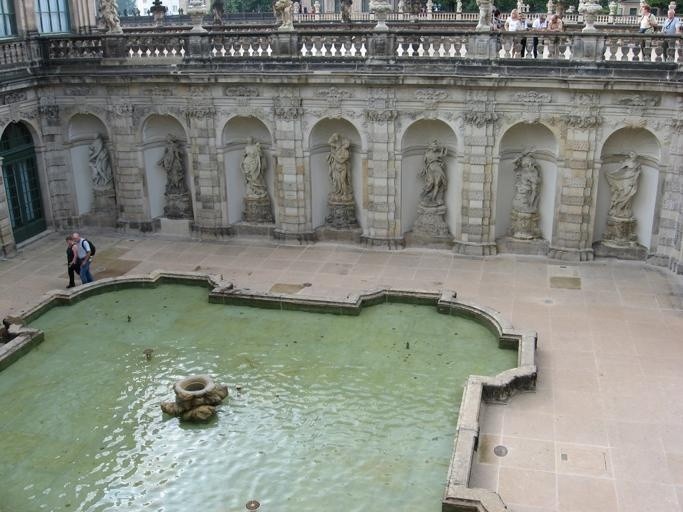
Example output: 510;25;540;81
67;272;75;288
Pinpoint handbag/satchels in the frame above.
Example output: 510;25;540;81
82;240;96;256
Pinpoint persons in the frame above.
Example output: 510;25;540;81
65;236;81;288
511;143;542;213
494;9;563;61
123;7;150;18
72;232;93;283
239;136;268;199
416;137;449;207
326;132;355;203
87;132;114;186
638;5;660;47
661;10;680;43
605;151;642;218
155;134;188;195
